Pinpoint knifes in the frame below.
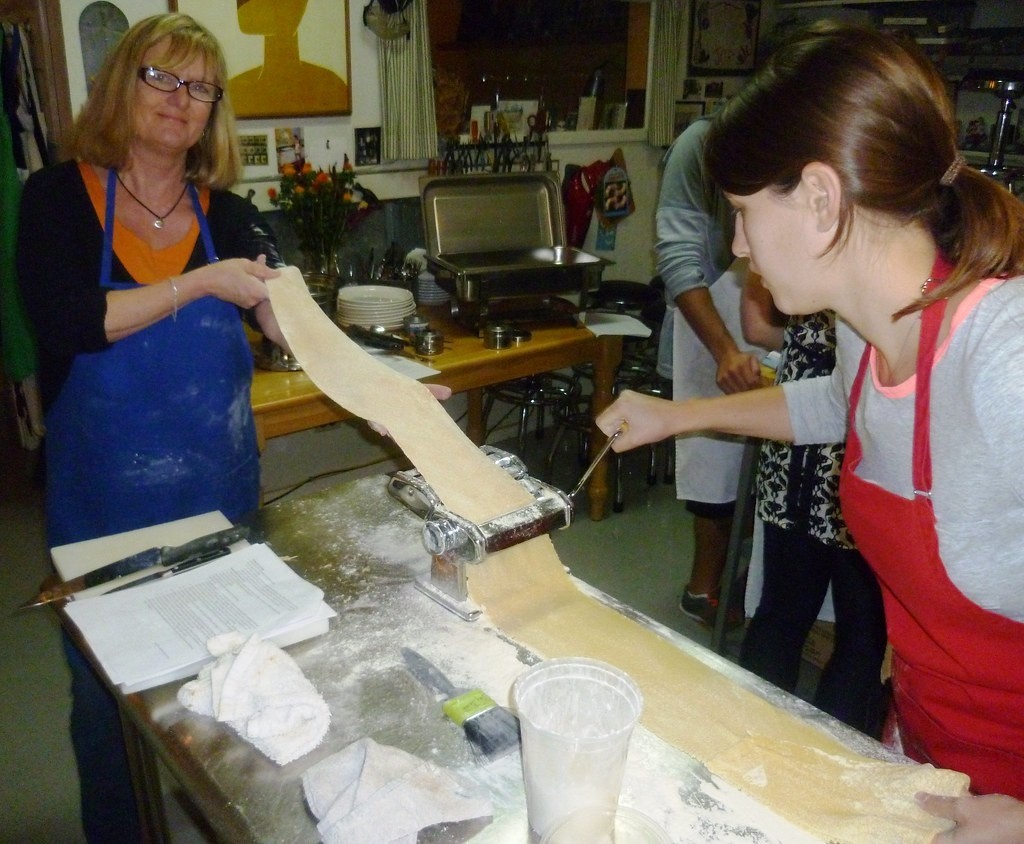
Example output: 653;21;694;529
19;524;250;609
101;547;231;596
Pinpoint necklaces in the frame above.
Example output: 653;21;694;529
113;174;190;229
876;314;919;387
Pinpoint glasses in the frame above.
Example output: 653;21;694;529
137;66;224;106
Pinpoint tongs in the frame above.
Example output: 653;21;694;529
335;322;405;352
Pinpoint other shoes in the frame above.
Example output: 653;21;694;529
680;581;744;633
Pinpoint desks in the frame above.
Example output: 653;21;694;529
246;303;625;518
32;475;974;844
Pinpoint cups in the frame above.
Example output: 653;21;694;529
510;657;644;840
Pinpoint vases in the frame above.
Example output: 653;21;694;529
313;253;343;319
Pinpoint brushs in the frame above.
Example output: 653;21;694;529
402;646;520;762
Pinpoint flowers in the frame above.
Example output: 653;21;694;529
266;152;380;276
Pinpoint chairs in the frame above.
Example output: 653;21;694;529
479;276;675;511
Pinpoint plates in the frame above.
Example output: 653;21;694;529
337;285;417;332
359;333;404;352
418;269;451;304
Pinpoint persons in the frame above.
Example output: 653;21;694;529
594;26;1023;844
15;12;451;843
653;113;791;631
736;277;891;740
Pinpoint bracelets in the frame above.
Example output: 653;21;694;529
169;277;179;322
